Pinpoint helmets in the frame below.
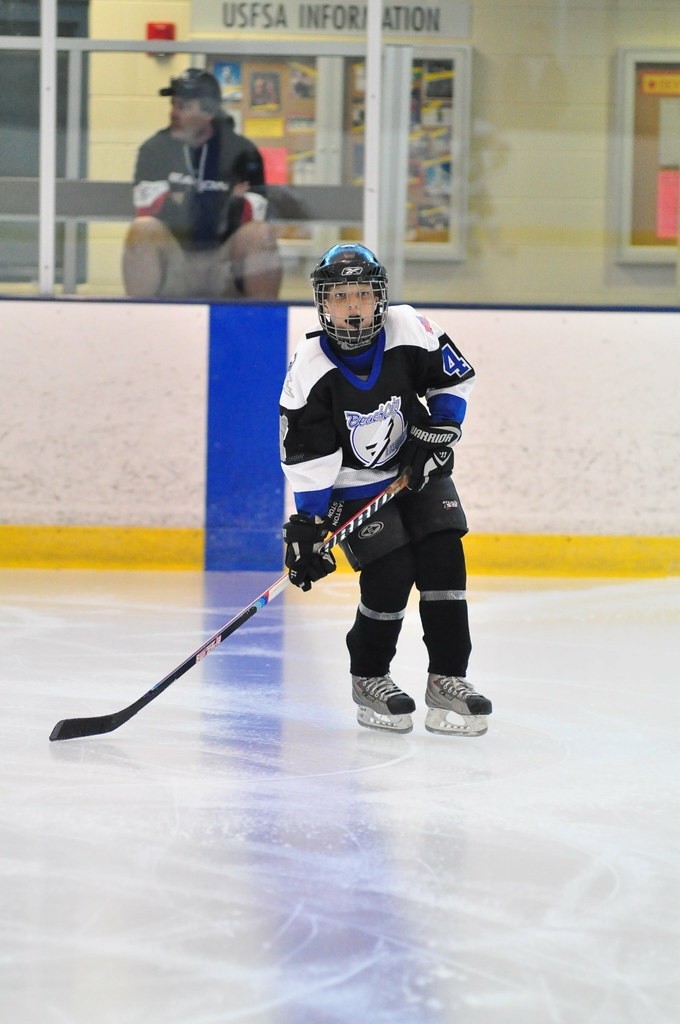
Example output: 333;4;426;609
309;242;388;351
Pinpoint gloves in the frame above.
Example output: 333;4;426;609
282;514;336;591
397;418;462;490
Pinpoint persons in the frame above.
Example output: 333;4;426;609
122;67;283;301
276;243;493;736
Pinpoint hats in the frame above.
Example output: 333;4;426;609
159;67;220;101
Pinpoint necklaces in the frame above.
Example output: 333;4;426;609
182;140;208;194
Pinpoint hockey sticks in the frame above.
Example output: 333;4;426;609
50;469;409;744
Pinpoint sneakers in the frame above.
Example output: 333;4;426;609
352;671;416;734
425;673;492;737
